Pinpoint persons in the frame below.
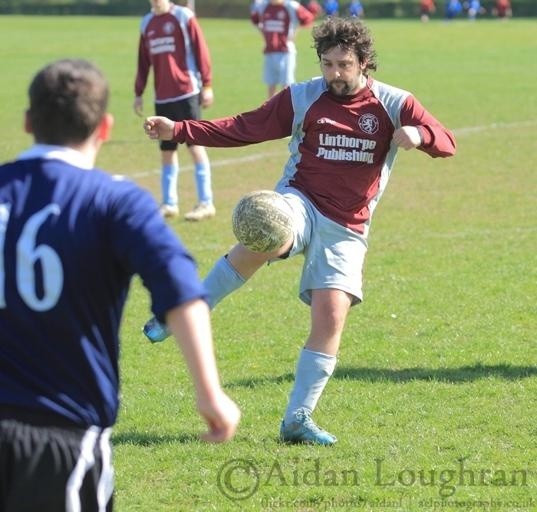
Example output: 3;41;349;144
130;1;215;220
3;59;239;509
249;1;313;99
137;15;460;447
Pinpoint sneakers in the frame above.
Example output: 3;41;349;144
280;413;336;445
184;203;215;221
143;317;170;342
159;204;179;218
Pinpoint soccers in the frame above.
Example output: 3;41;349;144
232;191;293;252
